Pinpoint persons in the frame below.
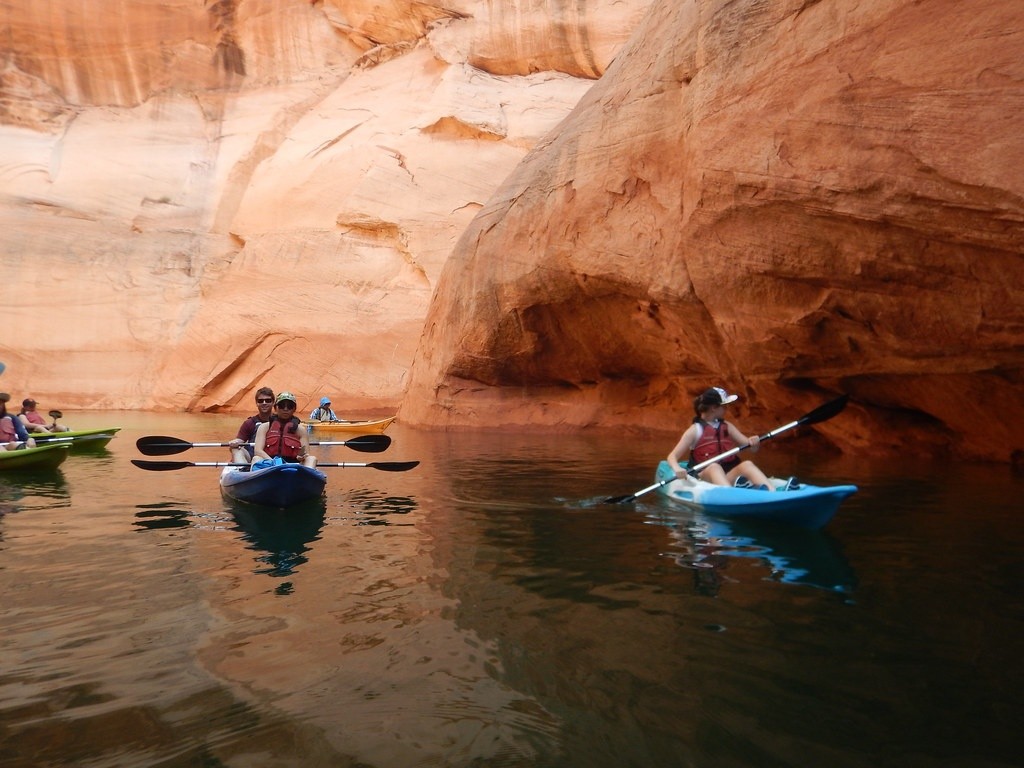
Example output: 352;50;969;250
228;387;275;473
17;398;72;433
306;397;338;435
668;387;800;492
251;392;317;473
0;393;36;452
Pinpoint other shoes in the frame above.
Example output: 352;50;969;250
16;444;26;450
776;476;800;491
759;484;769;490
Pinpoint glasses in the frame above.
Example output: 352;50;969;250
257;399;272;403
277;403;294;410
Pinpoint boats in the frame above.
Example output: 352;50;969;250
27;427;122;450
652;459;857;534
0;443;74;474
217;463;327;508
304;415;398;435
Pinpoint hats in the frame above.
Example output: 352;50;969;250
23;398;39;407
0;392;10;402
702;386;738;405
320;397;331;407
275;392;297;405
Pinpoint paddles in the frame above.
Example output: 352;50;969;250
0;434;118;446
49;410;63;432
602;390;848;505
301;419;379;424
131;459;420;473
137;434;392;456
